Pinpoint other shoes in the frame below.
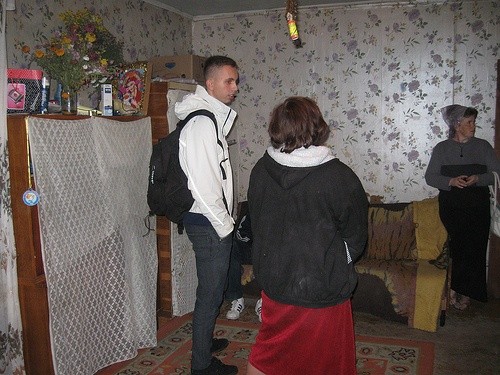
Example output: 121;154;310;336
209;338;229;353
190;357;238;375
446;293;471;311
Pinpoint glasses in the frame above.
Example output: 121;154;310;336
460;121;477;126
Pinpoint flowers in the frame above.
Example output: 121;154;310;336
21;7;128;107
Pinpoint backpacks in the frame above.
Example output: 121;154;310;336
146;109;219;225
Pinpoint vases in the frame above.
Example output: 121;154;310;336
60;83;78;115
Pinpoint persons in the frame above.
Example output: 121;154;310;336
174;55;263;375
244;96;369;375
424;104;500;311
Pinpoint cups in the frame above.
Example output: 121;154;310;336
49;100;60;111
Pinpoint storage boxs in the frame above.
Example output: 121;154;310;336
135;55;208;85
100;84;113;116
113;61;153;116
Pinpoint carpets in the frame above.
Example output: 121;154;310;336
92;309;436;375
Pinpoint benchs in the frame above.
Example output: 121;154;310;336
235;199;452;331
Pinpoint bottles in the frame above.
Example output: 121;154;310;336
41;75;51;114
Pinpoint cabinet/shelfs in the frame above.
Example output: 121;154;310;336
146;80;199;318
8;114;157;375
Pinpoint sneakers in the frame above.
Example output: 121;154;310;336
227;298;245;320
254;298;262;323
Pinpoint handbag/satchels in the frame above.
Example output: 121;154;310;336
485;172;500;238
